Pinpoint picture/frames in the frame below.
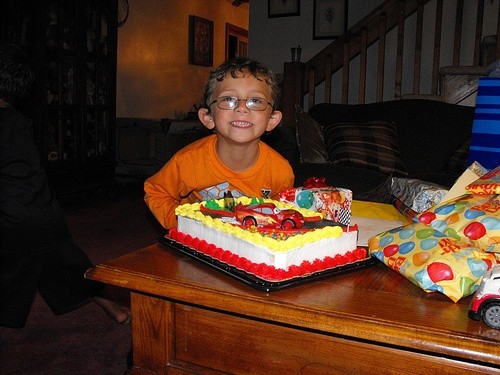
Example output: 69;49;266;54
268;0;300;19
312;0;349;41
188;15;214;67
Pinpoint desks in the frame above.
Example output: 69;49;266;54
83;242;500;375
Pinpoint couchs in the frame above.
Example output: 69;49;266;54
263;99;475;205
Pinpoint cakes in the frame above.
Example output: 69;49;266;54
167;196;366;282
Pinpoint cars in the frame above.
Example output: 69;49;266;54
468;263;499;330
234;202;305;230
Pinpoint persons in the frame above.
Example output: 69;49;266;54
0;43;128;329
143;56;296;232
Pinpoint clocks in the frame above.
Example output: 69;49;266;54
102;0;130;27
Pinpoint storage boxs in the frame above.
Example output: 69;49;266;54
279;185;353;226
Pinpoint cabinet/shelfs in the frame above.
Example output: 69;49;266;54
0;0;118;209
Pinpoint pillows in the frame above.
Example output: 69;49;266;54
468;77;500;169
294;103;330;164
324;121;409;175
448;138;471;175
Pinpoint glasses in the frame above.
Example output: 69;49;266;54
208;95;274;112
